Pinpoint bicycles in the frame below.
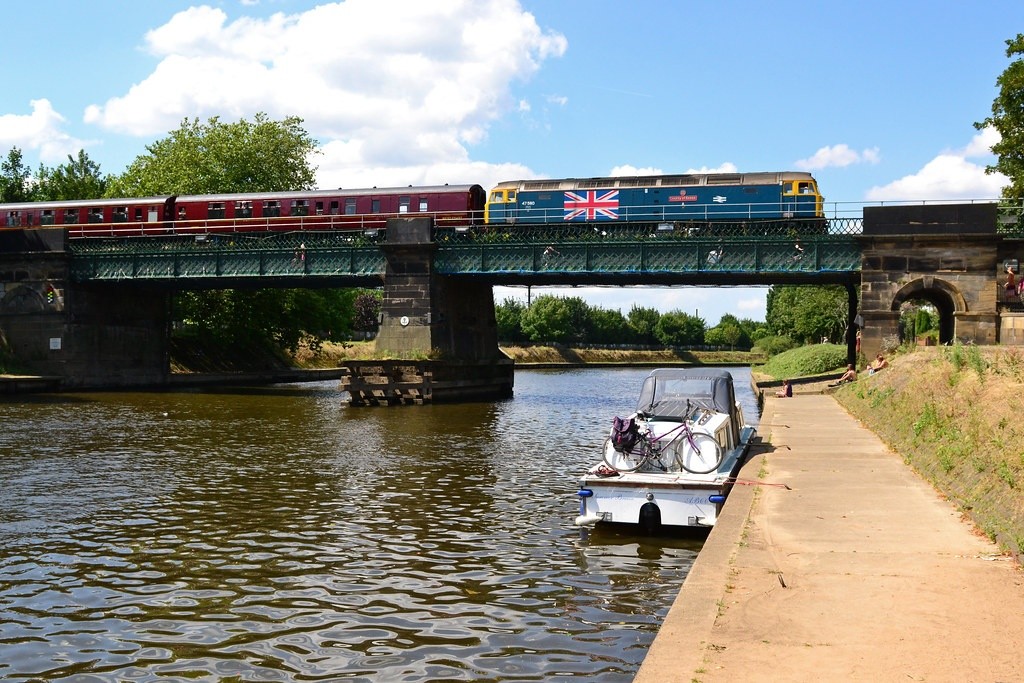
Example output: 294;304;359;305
601;397;724;474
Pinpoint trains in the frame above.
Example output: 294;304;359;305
0;170;826;248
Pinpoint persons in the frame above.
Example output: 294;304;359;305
823;337;828;344
1017;277;1024;294
778;380;788;397
856;331;860;352
840;364;857;382
873;354;889;372
1005;267;1016;290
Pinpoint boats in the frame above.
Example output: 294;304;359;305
575;368;758;534
